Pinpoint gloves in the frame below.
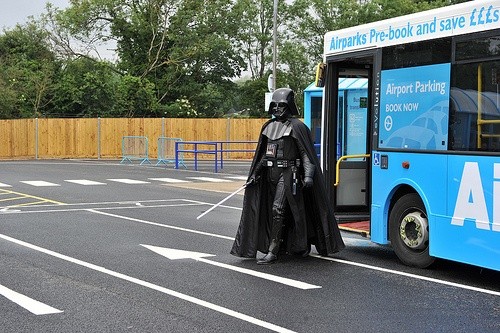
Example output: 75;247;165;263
250;162;263;184
303;155;314;188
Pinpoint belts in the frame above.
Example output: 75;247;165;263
263;160;300;167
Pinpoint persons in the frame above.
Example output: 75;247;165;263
230;88;346;265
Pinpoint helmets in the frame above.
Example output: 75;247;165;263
269;88;299;116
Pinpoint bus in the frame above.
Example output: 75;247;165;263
315;0;500;272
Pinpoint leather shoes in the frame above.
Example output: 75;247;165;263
256;252;279;265
